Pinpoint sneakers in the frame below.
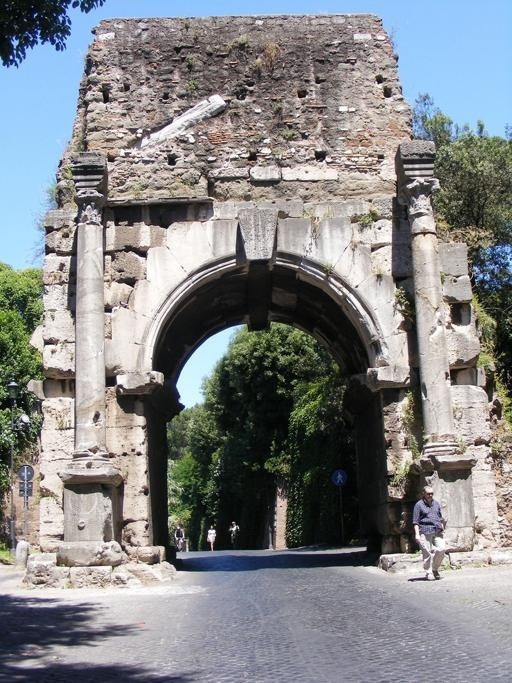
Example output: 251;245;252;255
425;569;440;581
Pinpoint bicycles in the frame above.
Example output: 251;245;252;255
175;537;184;552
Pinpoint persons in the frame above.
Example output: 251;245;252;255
228;521;240;549
413;486;446;581
175;526;184;550
207;525;216;551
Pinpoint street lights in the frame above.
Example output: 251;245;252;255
7;375;21;554
